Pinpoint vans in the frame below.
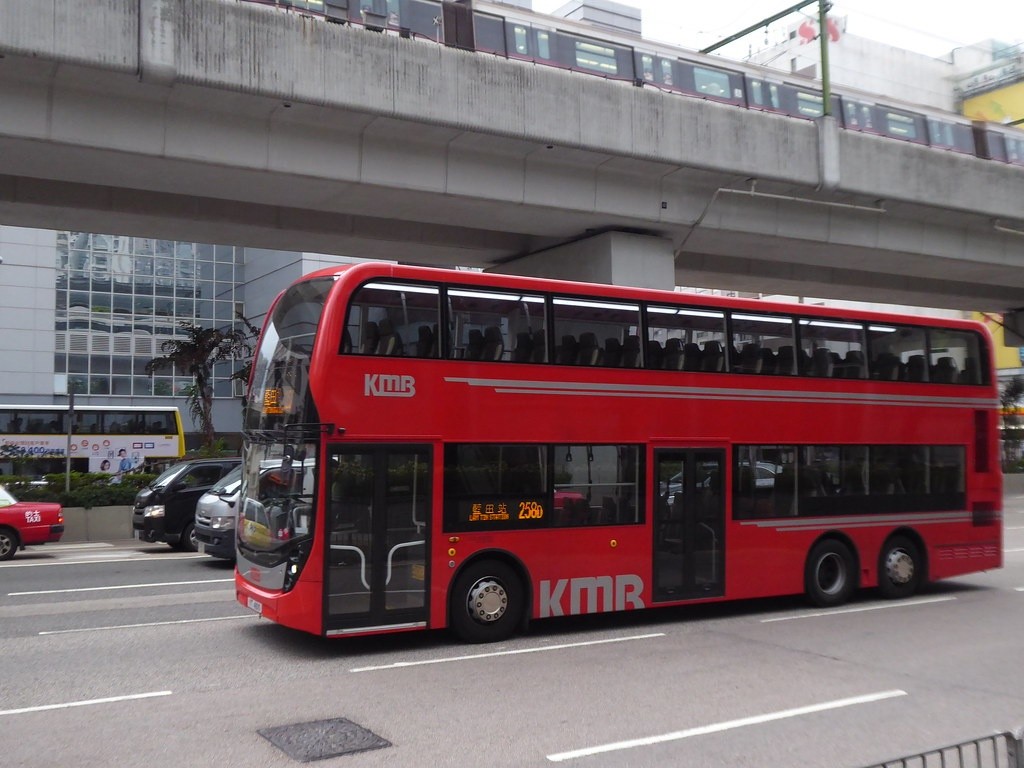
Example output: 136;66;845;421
191;453;340;566
131;455;243;551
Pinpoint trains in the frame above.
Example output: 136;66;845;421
261;0;1023;170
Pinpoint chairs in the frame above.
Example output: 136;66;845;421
361;320;982;386
453;459;962;530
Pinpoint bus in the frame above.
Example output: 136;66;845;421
228;261;1007;645
1;404;186;485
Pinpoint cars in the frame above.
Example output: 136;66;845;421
0;486;64;565
